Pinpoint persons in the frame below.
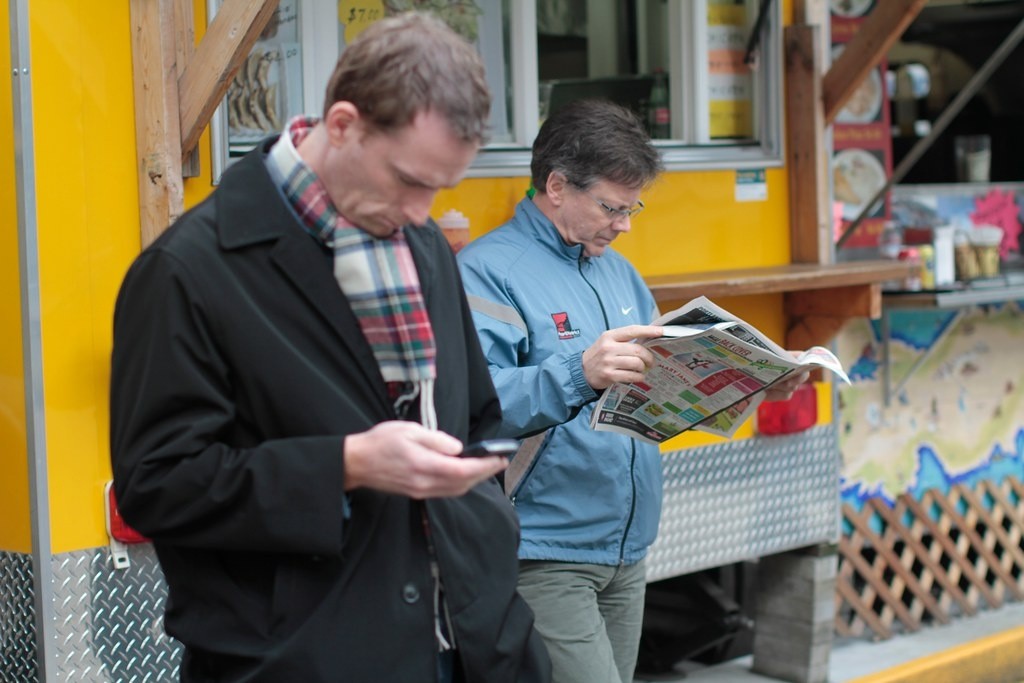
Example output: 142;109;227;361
108;8;557;683
453;99;810;683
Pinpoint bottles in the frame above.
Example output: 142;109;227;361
878;220;903;293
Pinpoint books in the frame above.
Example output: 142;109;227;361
588;295;852;447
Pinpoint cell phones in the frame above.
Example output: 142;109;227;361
459;437;520;457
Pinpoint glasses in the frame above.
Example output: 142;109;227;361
565;178;645;222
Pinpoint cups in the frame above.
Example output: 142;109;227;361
953;134;991;183
